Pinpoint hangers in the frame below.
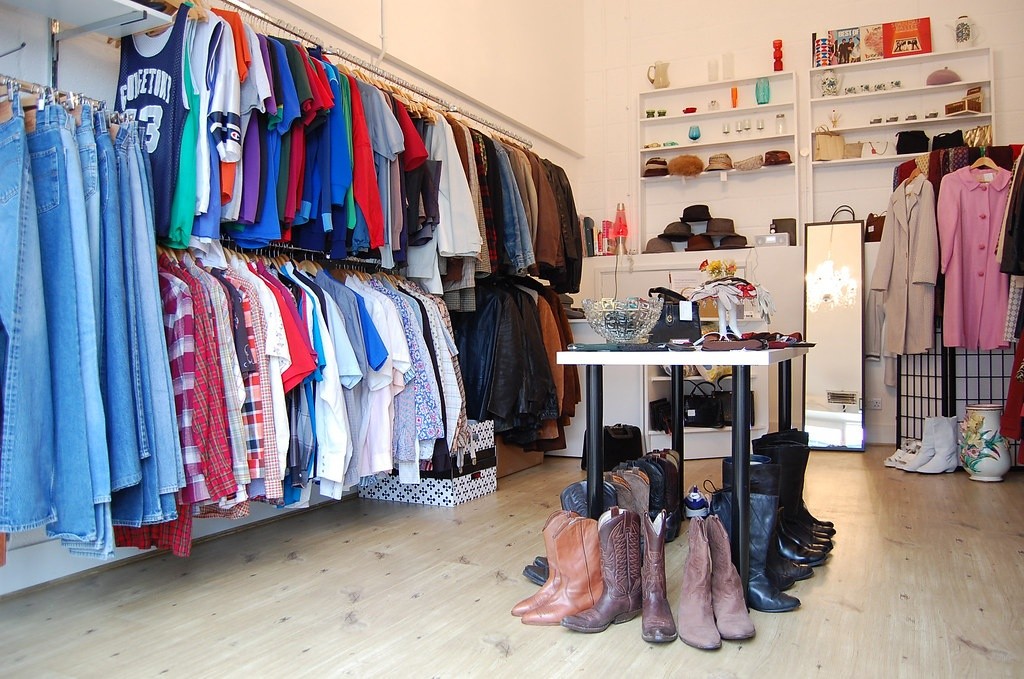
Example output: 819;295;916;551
108;0;533;152
155;234;408;289
0;74;134;127
903;166;921;195
969;148;998;183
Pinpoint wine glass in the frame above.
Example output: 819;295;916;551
722;120;764;137
689;125;701;144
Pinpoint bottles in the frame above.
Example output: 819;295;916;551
777;114;786;135
709;101;719;110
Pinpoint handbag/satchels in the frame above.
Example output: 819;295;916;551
681;379;725;429
648;287;702;346
711;374;755;427
580;424;643;471
830;203;887;243
812;124;992;161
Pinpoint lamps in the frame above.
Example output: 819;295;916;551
826;391;856;404
806;225;856;313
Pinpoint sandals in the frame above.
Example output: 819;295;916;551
884;449;904;468
896;444;920;471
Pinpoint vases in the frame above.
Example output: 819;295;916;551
960;404;1011;482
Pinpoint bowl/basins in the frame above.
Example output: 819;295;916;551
582;299;664;343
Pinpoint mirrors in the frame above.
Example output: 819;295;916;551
801;219;865;452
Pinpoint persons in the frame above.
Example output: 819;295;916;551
839;38;854;64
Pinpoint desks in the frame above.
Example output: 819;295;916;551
555;341;809;605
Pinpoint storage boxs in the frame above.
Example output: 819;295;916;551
450;419;494;466
357;457;497;508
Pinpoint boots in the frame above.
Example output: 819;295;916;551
917;415;960;473
510;429;838;651
904;417;935;471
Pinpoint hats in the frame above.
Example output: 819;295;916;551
643;151;792;178
642;205;756;254
927;66;960;85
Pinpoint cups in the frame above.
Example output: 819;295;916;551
755;78;769;104
722;54;733;78
647;62;670;88
708;60;718;81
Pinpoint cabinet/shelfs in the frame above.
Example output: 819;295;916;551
636;71;802;460
808;46;998;244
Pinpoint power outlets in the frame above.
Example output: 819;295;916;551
865;398;882;410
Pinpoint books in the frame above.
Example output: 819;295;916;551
585;217;614;257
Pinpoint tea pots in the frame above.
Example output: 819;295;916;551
944;15;979;48
